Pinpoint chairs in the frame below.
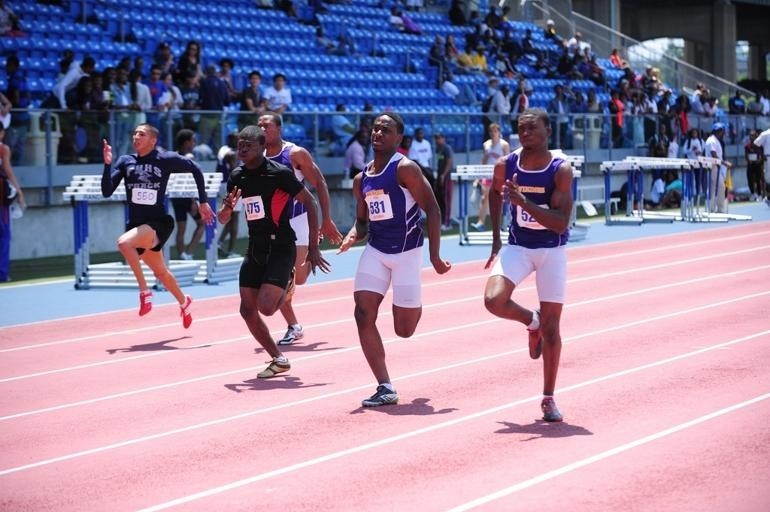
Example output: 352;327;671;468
0;0;154;106
486;20;626;112
155;0;487;153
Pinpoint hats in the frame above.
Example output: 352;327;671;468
555;84;562;88
713;123;724;131
489;76;498;83
249;71;261;77
273;73;287;82
160;41;171;48
435;132;444;137
219;58;235;69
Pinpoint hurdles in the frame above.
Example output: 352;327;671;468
450;155;592;246
61;171;245;292
598;155;753;226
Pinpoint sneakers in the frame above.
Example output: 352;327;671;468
227;251;241;258
277;323;304;345
440;223;453;230
258;357;291;378
217;244;227;256
527;308;545;359
757;196;762;201
541;397;563;422
754;193;758;199
750;194;754;201
179;295;193;328
471;222;485;231
139;292;153;316
180;251;194;259
362;385;399;406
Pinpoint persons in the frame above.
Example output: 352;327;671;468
217;58;242;103
91;71;110;111
5;55;31;151
111;65;131;147
430;2;719;147
157;40;177;70
0;91;11;141
141;65;175;146
134;55;145;79
39;55;95;112
397;11;426;35
470;123;511;235
316;27;360;58
752;128;770;205
256;114;343;346
747;91;765;115
179;69;199;110
705;122;732;212
265;74;292;125
682;128;705;159
237;71;265;131
118;68;152;156
411;128;436;181
434;131;456;232
116;56;131;71
65;50;78;68
198;66;228;149
728;90;745;115
398;132;410;157
64;76;92;110
171;127;201;261
58;60;68;81
104;67;116;90
745;131;763;203
0;1;26;37
337;111;453;412
177;40;207;86
217;125;332;380
648;124;669;178
361;116;372;136
217;129;241;260
161;72;184;129
0;122;26;283
102;123;218;328
345;129;368;179
362;98;373;112
389;6;419;36
650;171;665;203
484;106;572;421
658;171;685;209
332;105;356;136
